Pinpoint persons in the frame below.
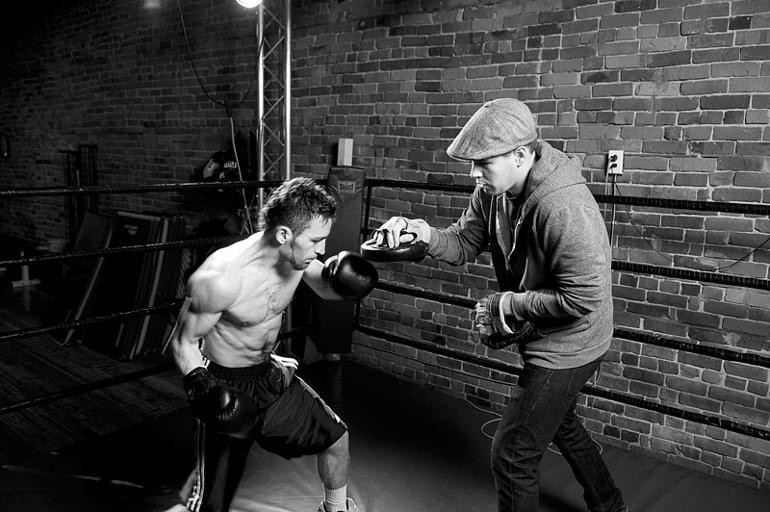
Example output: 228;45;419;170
160;175;379;512
367;98;627;512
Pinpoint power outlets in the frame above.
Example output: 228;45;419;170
606;149;624;177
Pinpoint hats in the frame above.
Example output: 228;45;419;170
446;98;538;161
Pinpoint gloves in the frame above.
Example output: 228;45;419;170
320;249;378;302
183;368;242;428
